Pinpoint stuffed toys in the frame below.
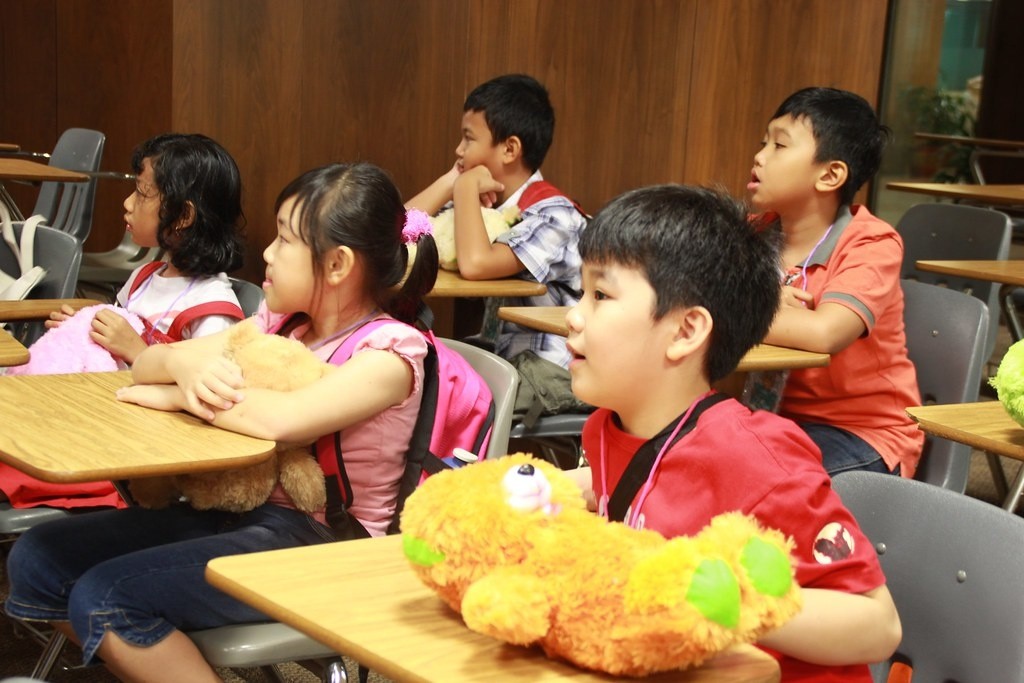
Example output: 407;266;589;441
7;300;143;372
403;195;519;270
130;316;329;518
400;451;805;678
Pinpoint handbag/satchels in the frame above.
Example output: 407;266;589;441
0;201;46;326
510;350;593;470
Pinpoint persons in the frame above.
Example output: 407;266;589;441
564;182;902;683
4;75;588;683
745;87;925;479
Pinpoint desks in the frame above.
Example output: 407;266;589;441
914;132;1024;185
887;180;1024;211
203;531;782;683
0;299;106;368
497;305;832;475
389;256;550;355
0;370;277;502
916;259;1024;342
0;157;91;221
905;402;1024;515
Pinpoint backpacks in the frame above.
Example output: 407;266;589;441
265;311;496;542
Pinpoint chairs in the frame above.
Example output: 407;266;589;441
0;127;1024;683
